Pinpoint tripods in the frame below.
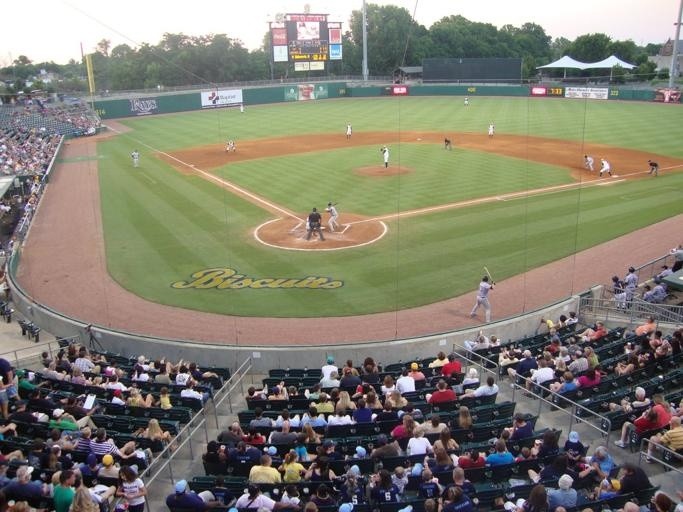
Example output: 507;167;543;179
89;332;104;351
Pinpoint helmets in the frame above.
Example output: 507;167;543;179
481;276;488;282
612;276;618;282
629;267;634;272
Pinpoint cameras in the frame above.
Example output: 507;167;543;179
84;323;91;332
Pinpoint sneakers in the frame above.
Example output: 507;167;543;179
614;440;625;448
641;453;653;464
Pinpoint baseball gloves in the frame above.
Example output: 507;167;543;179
381;148;383;151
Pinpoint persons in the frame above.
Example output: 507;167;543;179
463;95;468;108
465;311;682;465
583;155;594;173
470;275;495;323
165;351;682;512
0;244;10;298
612;245;682;314
304;208;325;242
130;148;140;168
487;121;495;140
1;137;62;244
240;101;244;113
443;136;451;151
296;22;307;38
0;91;100;138
647;159;658;178
344;122;352;141
598;158;613;178
0;335;221;512
379;146;389;169
323;201;339;233
224;140;236;153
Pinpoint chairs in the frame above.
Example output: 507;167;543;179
3;348;233;511
452;321;683;474
164;355;682;512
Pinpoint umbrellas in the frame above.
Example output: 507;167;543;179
585;54;638;83
535;55;584;81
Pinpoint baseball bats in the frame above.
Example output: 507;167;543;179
483;265;496;283
328;202;338;208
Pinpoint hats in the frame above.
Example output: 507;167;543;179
327;357;334;364
115;503;128;512
271;387;279;392
53;408;65;418
569;431;579;443
175;479;187;494
15;370;25;378
611;480;621;491
661;265;667;269
268;446;277;456
323;439;338;449
113;390;121;397
558;474;574;490
411;363;418;370
85;454;97;466
103;454;113;465
17;466;34;481
569;312;577;317
506;351;515;359
339;503;353;512
356;446;366;457
644;285;650;290
